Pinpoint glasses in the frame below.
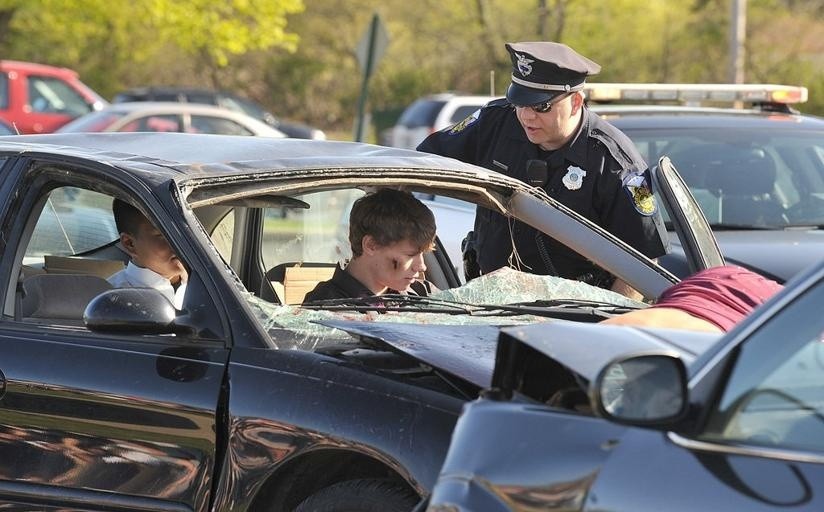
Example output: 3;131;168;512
511;92;575;114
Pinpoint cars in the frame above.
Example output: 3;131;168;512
563;82;823;280
393;92;505;148
51;100;288;137
116;87;324;140
421;265;824;511
0;136;738;509
1;60;108;134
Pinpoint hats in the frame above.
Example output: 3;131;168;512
503;40;601;105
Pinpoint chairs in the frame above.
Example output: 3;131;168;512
11;252;126;329
705;143;786;230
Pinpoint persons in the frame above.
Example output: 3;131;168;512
591;264;792;338
297;185;439;308
411;37;672;304
105;194;189;312
